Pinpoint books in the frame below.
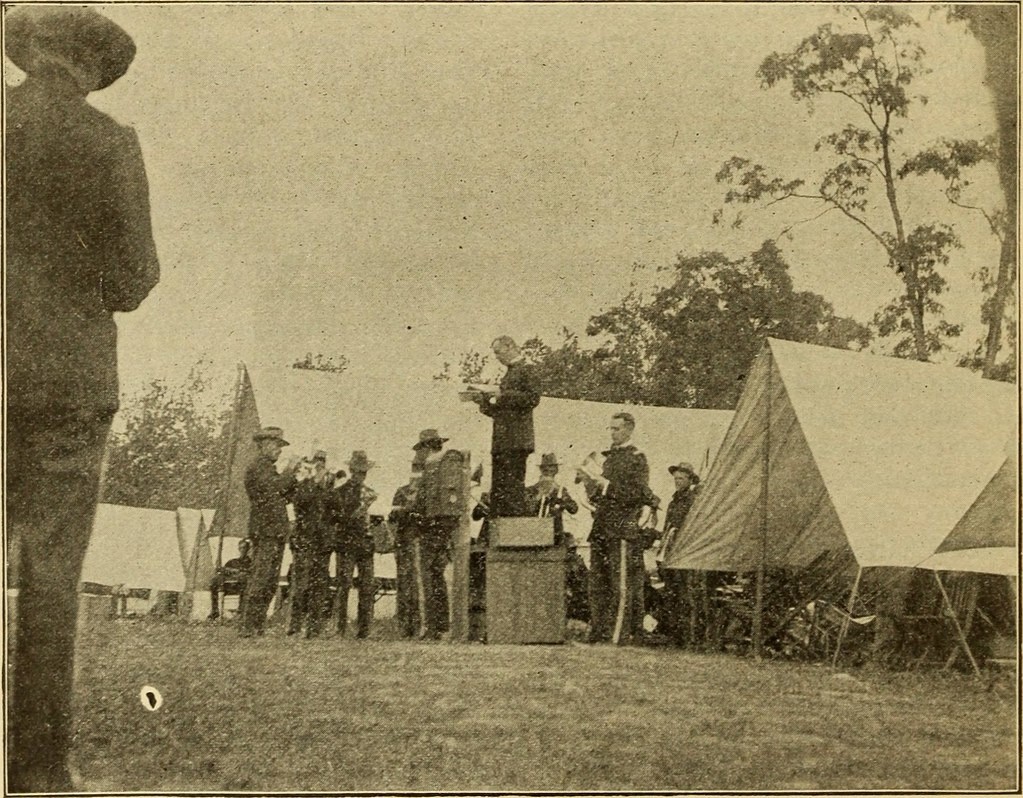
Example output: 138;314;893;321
578;456;603;481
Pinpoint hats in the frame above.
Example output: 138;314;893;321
537;452;562;466
668;462;699;485
253;426;291;447
413;429;449;451
345;450;374;472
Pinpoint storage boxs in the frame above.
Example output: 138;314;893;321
486;517;554;548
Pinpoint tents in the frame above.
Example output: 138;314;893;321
185;370;738;623
661;341;1018;668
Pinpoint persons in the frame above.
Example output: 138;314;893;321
207;424;469;641
660;461;700;652
4;6;161;794
473;491;491;589
562;533;590;632
472;334;540;517
575;412;649;646
524;453;579;546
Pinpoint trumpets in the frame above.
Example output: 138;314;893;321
277;451;348;489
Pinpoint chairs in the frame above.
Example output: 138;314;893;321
902;574;983;670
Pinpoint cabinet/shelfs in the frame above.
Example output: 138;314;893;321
468;544;567;644
